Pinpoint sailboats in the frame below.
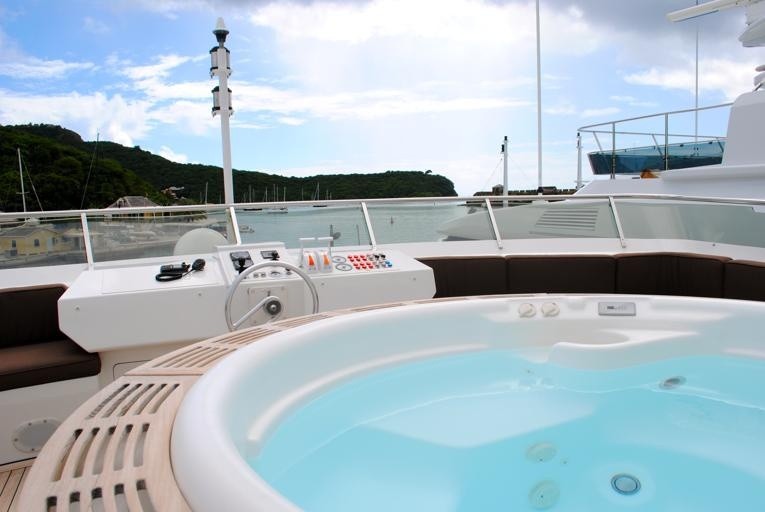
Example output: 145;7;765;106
237;180;334;213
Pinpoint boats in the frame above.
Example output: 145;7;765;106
439;0;765;254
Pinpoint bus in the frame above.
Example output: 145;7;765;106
124;231;158;242
102;224;134;239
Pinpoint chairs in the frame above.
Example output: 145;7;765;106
0;285;103;469
